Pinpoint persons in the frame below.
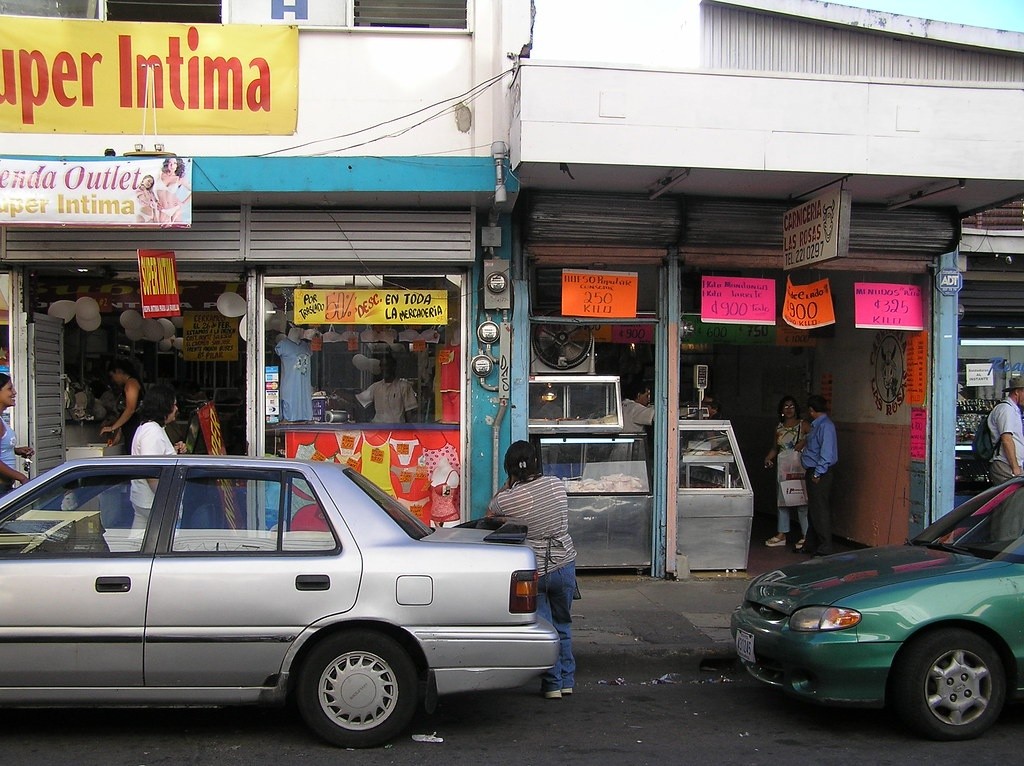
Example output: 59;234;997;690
136;175;162;225
765;396;811;548
128;385;187;530
99;357;146;527
801;395;839;558
275;327;314;422
487;440;582;699
987;376;1024;541
156;158;191;224
608;383;730;458
321;356;419;424
0;373;35;499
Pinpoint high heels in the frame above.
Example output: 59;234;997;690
766;536;785;547
795;538;806;548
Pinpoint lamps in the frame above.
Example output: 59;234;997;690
887;182;967;212
650;169;692;200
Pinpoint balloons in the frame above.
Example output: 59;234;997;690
141;318;164;341
157;319;175;339
159;338;171;352
264;300;273;323
239;314;247;341
74;296;100;320
48;299;76;324
217;292;246;318
124;328;144;340
76;313;101;331
174;338;183;349
120;309;142;329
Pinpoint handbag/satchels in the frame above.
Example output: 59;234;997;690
777;449;806;483
777;477;809;507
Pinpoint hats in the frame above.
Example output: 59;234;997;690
1002;376;1024;392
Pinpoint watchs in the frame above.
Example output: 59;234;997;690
813;474;819;479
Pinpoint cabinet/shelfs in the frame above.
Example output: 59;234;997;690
679;419;752;492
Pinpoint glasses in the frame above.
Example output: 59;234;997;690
172;402;178;408
784;405;795;409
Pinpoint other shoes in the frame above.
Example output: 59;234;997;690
544;690;562;698
811;552;831;558
562;688;573;694
793;548;814;554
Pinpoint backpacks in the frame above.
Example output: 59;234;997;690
971;399;1015;475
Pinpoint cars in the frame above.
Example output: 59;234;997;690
0;455;561;748
730;474;1024;742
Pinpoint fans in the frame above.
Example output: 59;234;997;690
532;306;598;377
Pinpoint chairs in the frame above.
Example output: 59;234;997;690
189;503;226;531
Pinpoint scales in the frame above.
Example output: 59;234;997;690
680;365;710;419
